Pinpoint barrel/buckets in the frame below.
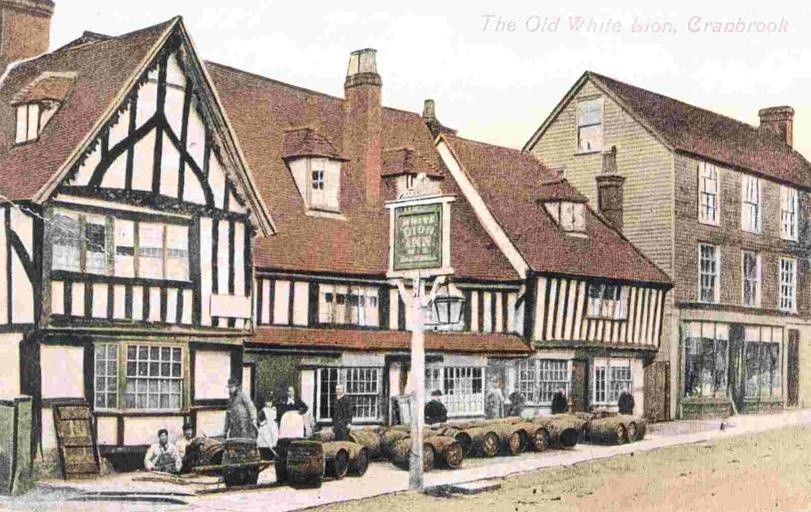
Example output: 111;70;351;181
305;410;646;461
184;435;220;466
275;437;325;488
222;439;261;488
391;436;465;471
324;441;370;480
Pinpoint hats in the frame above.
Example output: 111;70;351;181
183;423;193;428
225;377;240;387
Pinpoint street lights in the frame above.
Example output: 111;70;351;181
405;275;466;491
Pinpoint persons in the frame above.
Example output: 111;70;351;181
551;388;570;414
485;378;526;418
143;377;355;473
422;390;448;424
618;387;635;415
